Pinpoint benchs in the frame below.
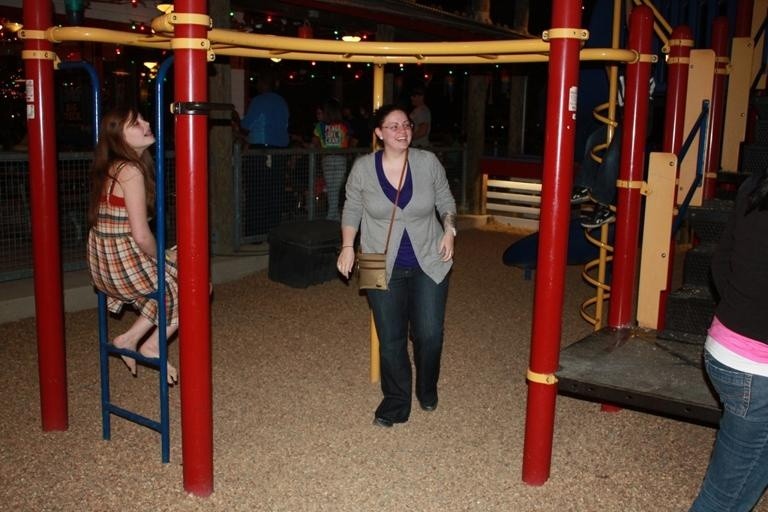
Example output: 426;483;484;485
554;325;721;427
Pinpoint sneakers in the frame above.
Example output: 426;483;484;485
571;187;590;204
420;400;438;410
376;417;408;427
580;206;614;228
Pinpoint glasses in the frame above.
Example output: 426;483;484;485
381;123;413;131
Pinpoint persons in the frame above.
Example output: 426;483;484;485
231;72;432;245
686;163;768;512
11;118;86;242
85;103;214;387
335;103;459;429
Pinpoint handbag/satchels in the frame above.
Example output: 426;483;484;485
357;252;387;291
